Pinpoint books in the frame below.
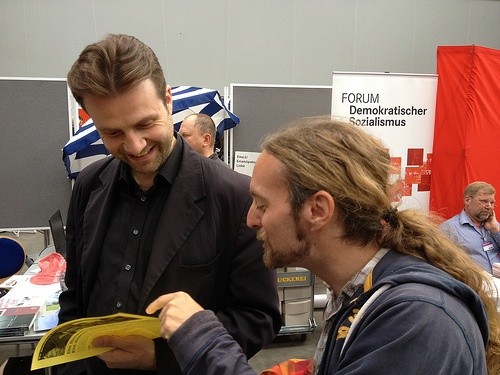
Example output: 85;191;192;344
0;274;68;343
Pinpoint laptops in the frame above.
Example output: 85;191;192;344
49;211;69;260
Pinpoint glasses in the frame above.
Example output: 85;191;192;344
468;195;497;206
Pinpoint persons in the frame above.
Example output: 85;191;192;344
435;180;500;278
145;118;489;375
179;111;233;170
55;32;282;375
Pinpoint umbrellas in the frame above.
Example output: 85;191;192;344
60;85;240;181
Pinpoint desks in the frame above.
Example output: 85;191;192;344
0;244;68;375
